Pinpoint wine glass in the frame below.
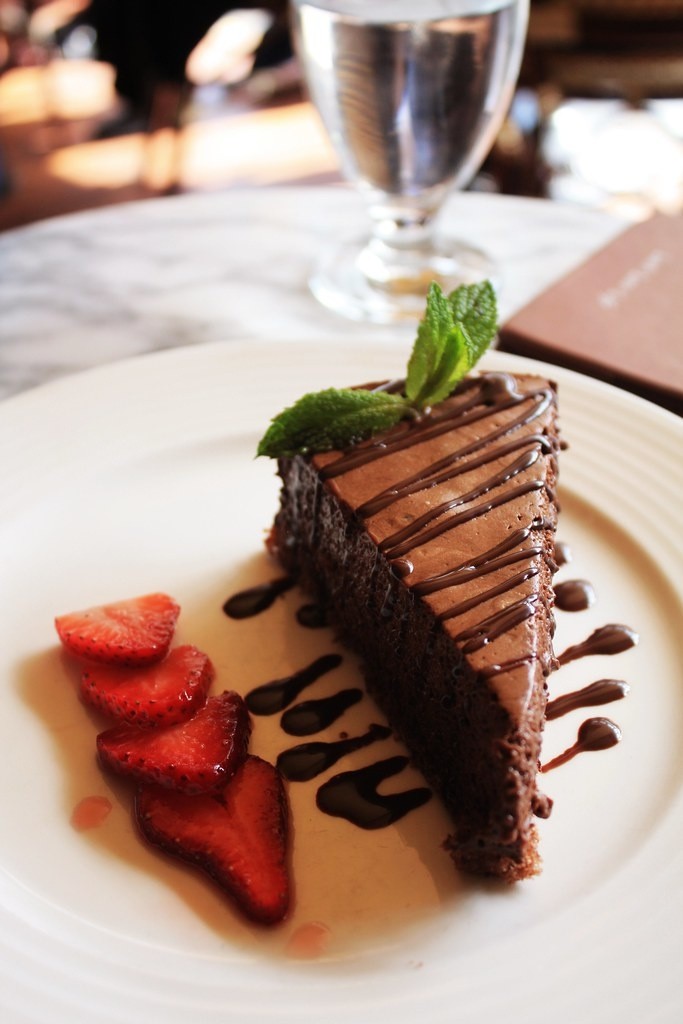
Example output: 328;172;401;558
293;0;531;325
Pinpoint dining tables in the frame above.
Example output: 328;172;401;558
0;188;638;398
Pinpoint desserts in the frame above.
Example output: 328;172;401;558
250;278;562;881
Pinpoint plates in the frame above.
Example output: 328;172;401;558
0;337;683;1024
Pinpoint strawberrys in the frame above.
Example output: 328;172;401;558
55;593;293;924
